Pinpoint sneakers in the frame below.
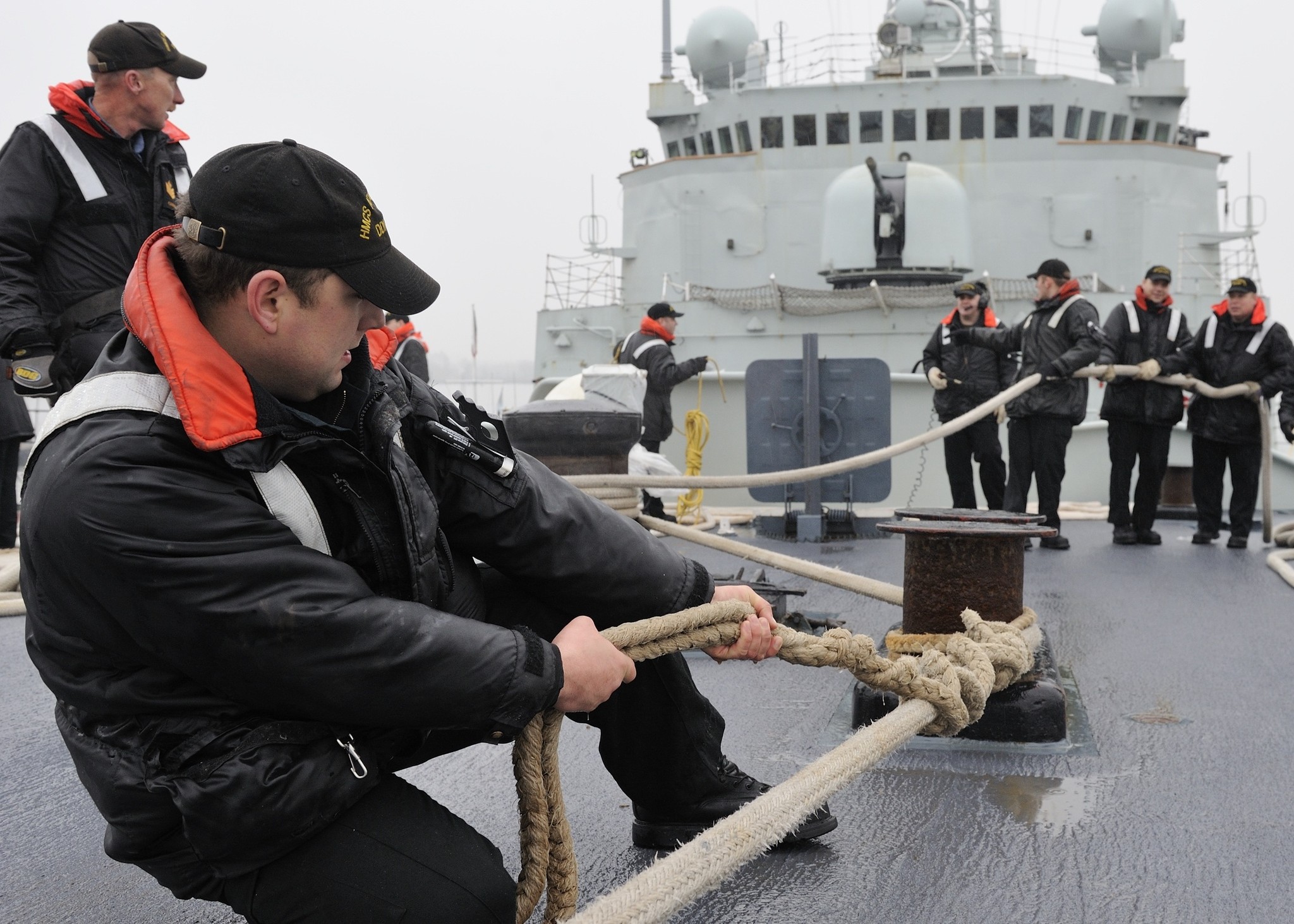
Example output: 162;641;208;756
632;755;838;846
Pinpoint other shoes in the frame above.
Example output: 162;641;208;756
1133;525;1161;544
1039;535;1070;548
1113;524;1138;544
1226;535;1248;548
661;515;677;524
1023;537;1031;548
1191;528;1220;544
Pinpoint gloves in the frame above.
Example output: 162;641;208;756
927;366;947;390
8;334;72;396
1095;363;1116;383
994;404;1006;425
945;329;969;347
1132;358;1161;381
1034;364;1058;388
1245;382;1262;405
696;356;708;372
1281;420;1294;442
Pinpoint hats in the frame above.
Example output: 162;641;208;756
648;303;685;319
87;19;207;80
1027;259;1071;279
1227;277;1257;293
185;139;440;315
953;283;981;296
1146;265;1171;281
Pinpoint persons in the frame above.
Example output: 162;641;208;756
922;258;1101;550
0;20;206;549
1095;265;1294;548
385;313;429;384
15;140;840;924
614;302;709;523
